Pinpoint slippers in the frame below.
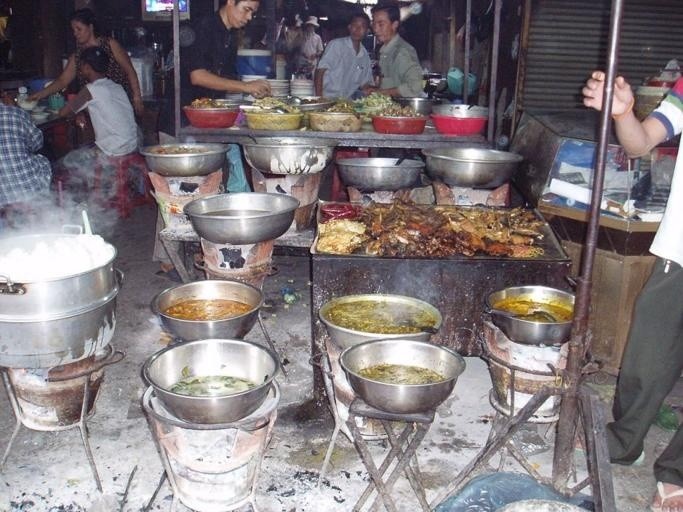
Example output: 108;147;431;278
573;429;645;465
651;481;683;512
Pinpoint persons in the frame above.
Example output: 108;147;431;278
578;69;682;512
51;44;144;193
149;0;271;290
310;11;376;100
0;101;52;210
288;15;324;78
23;7;146;151
357;1;425;98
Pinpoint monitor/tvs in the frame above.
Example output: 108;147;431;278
141;0;190;22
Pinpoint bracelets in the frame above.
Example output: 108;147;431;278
611;96;636;120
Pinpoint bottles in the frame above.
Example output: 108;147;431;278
110;18;174;98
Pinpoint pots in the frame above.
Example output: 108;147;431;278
0;223;125;369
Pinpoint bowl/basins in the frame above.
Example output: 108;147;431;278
142;337;281;425
137;141;232;177
421;146;525;190
150;279;265;345
332;156;426;192
182;96;488;136
237;136;340;178
485;283;593;346
338;338;466;415
318;292;443;350
182;192;301;245
12;87;77;121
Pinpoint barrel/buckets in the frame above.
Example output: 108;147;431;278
237;49;270;82
290;80;315;98
267;79;289;97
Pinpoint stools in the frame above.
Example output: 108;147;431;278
54;166;90;223
330;148;370;202
343;397;436;512
3;205;33;232
91;153;153;220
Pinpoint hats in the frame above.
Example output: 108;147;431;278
305;16;319;27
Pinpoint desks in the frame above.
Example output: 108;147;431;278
180;99;487;152
